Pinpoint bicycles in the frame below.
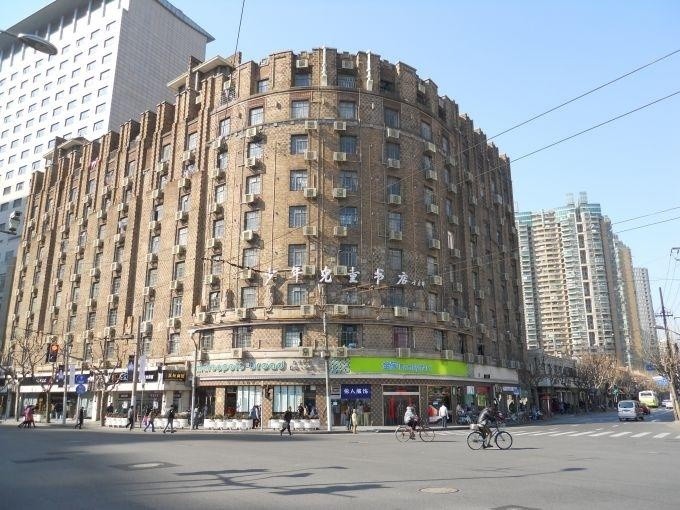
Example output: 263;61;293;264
395;417;435;443
466;417;513;450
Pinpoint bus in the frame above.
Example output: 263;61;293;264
639;391;659;408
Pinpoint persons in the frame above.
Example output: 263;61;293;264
477;405;498;449
17;401;200;435
250;403;365;438
438;403;449;428
403;404;420;439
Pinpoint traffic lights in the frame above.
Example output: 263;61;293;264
48;344;59;362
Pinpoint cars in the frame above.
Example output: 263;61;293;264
639;406;650;415
661;400;673;408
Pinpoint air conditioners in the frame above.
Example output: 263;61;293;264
304;119;318;132
385;157;404;170
332;224;351;239
222;79;234;90
332;302;350;319
394;345;413;360
303;187;318;200
233;307;251;321
209;166;223;180
422;140;527;370
331;187;349;199
202;273;218;286
239;229;258;243
329;345;350;361
301;263;317;281
195;310;210;325
209;137;225;153
392;305;411;320
228;347;245;360
295;346;316;360
238;268;255;282
241;192;257;205
14;146;196;370
205;237;218;250
294;58;311;73
244;127;262;143
437;99;446;109
333;265;348;277
332;151;348;165
333;120;348;134
241;156;259;169
418;83;427;95
387;193;404;206
302;149;319;163
298;303;315;319
383;126;402;140
207;200;219;213
388;229;405;242
340;58;357;73
301;224;319;238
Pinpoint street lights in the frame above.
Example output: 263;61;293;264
2;28;60;55
652;324;680;337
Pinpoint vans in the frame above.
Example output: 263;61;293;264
617;400;644;421
